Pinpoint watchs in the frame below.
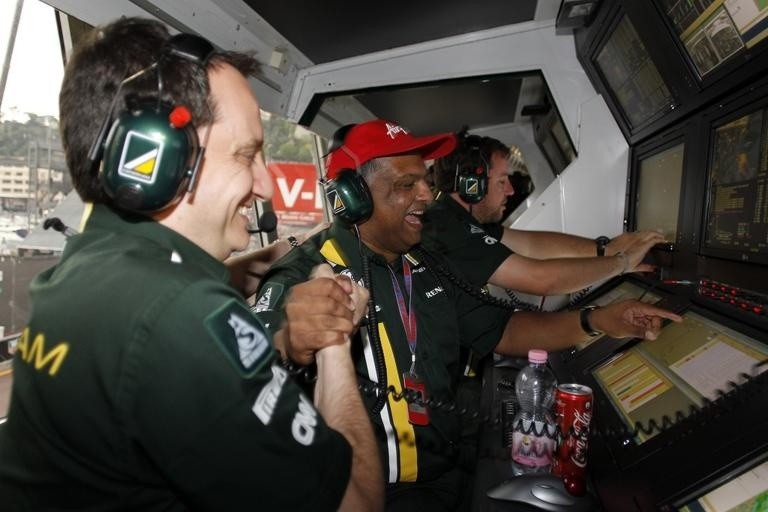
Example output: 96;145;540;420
594;235;608;256
578;302;602;340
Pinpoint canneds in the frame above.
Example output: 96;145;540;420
551;382;595;478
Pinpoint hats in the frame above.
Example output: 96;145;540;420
327;120;457;180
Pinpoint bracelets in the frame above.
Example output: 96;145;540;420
613;250;630;275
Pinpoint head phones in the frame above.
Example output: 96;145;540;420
453;134;489;205
319;122;375;228
84;33;215;224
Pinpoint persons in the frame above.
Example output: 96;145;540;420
2;13;388;510
251;110;681;512
417;126;672;442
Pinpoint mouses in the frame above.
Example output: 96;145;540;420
486;474;592;510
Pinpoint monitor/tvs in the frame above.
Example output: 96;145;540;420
660;443;768;511
547;115;577;165
588;7;677;135
653;0;768;89
631;134;686;247
586;303;768;452
698;96;768;267
536;133;565;177
571;280;666;354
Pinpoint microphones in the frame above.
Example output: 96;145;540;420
248;211;278;234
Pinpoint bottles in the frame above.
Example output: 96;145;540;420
510;349;558;476
